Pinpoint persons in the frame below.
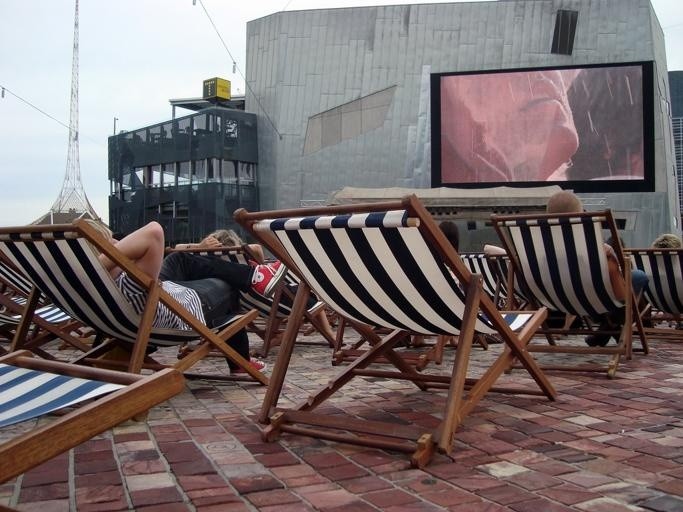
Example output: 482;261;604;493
652;232;682;332
439;69;580;184
606;234;625;255
75;215;290;378
546;191;648;347
438;220;510;346
173;229;340;344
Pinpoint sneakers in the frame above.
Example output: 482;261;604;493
231;357;268;377
584;336;606;347
251;260;289;298
